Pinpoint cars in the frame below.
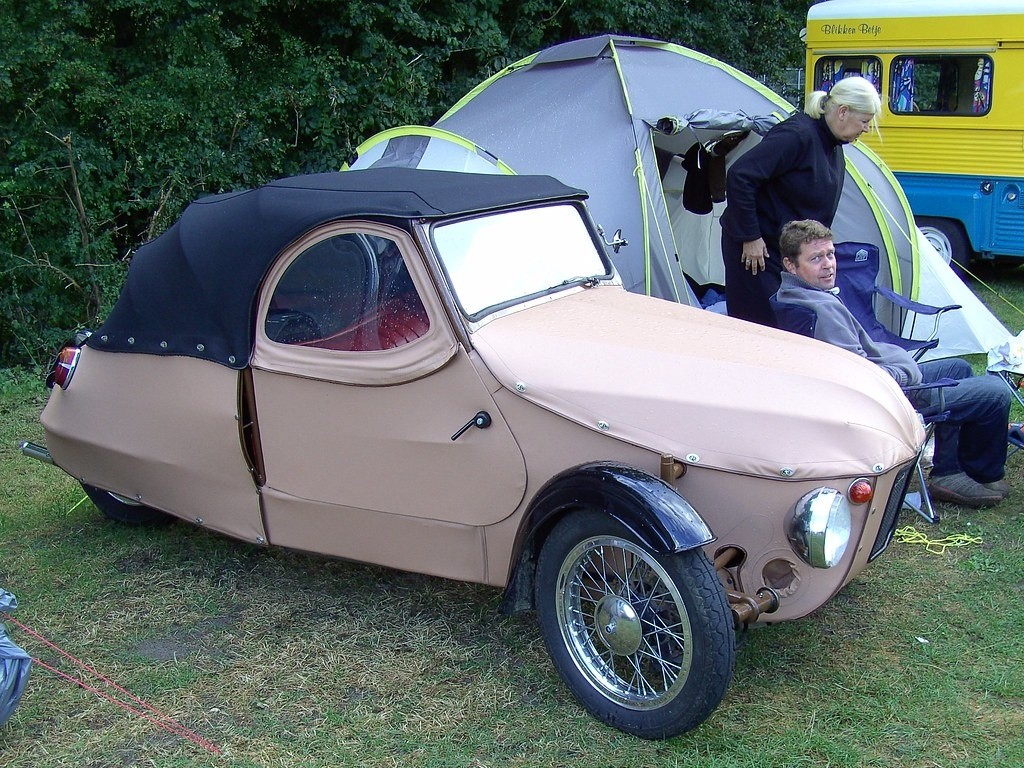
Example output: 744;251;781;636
26;171;936;743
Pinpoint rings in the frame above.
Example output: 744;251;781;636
746;260;750;262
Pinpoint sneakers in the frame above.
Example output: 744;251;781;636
929;472;1011;506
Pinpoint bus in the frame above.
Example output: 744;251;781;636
800;3;1024;281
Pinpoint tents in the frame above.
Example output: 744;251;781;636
338;37;1014;367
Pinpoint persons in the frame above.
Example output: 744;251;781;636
778;219;1011;505
719;77;883;328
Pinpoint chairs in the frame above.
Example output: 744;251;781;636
770;241;962;523
